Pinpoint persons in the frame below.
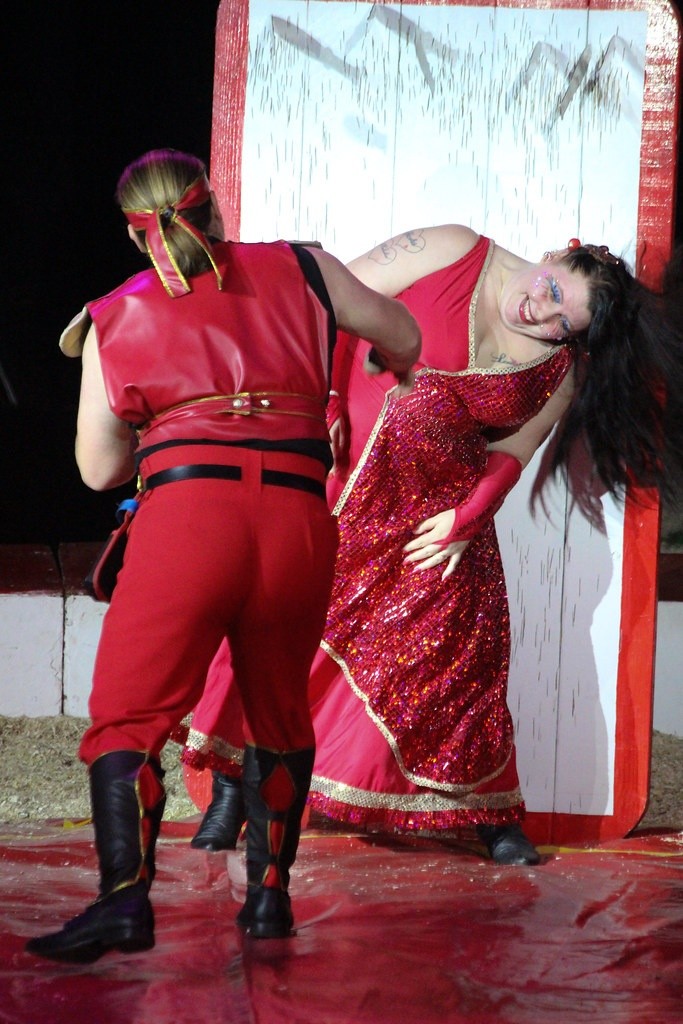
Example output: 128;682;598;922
182;225;683;864
23;152;421;964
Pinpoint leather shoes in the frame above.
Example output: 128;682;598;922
477;820;540;868
28;884;156;965
190;776;248;850
236;885;294;940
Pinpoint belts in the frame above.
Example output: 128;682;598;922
141;464;326;500
